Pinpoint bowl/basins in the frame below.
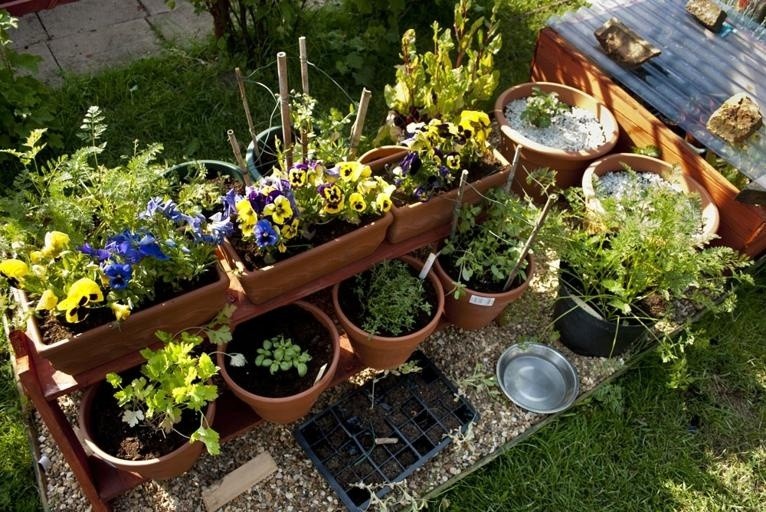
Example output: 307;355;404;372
495;342;581;416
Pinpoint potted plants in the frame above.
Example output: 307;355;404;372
330;254;447;368
494;82;622;203
581;152;722;252
430;169;570;331
555;172;759;389
78;309;237;481
216;300;341;424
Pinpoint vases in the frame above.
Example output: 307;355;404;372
364;144;513;253
247;121;330;182
148;160;242;194
14;245;231;375
216;207;395;304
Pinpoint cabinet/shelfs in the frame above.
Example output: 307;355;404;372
0;123;536;511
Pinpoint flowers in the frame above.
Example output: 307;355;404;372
393;111;500;201
230;157;397;250
1;108;239;326
